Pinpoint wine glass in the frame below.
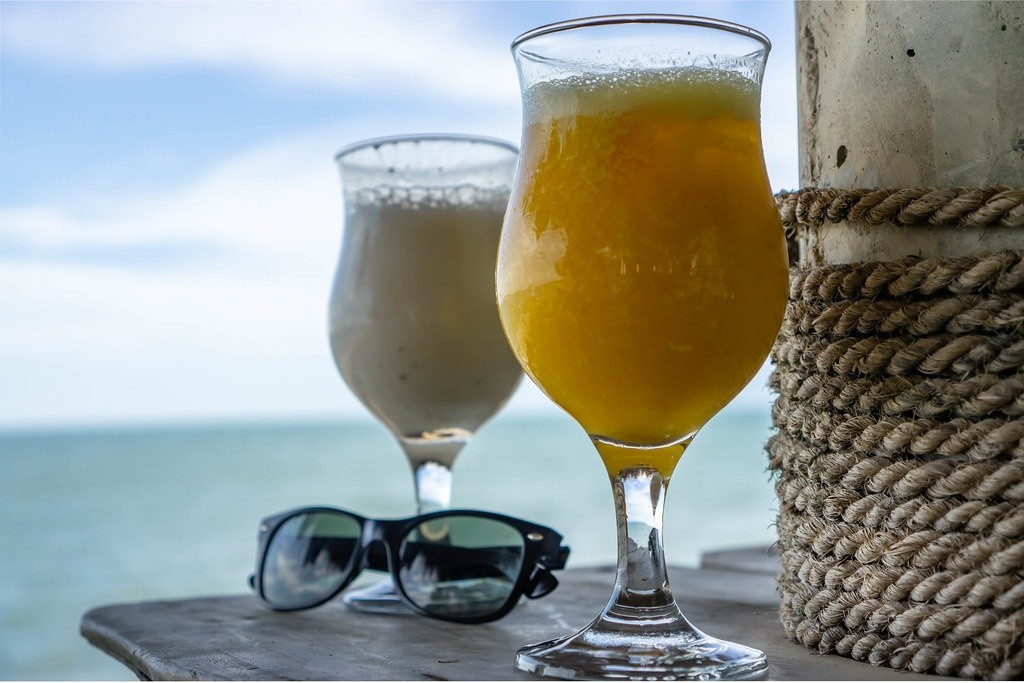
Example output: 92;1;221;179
495;13;787;681
326;133;526;616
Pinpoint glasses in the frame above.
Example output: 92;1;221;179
247;505;569;626
